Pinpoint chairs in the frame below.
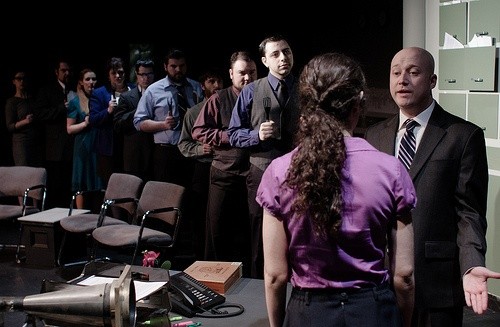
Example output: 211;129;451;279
58;173;142;273
0;166;47;265
66;181;185;283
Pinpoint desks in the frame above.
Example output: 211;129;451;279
18;207;91;267
89;264;293;327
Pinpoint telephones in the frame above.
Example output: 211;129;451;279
164;271;226;318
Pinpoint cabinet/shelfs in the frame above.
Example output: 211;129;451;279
438;0;500;297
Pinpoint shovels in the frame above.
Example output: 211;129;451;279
263;97;272;122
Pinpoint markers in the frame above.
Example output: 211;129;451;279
110;92;114;101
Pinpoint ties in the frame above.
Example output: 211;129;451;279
175;84;191;126
398;119;421;172
277;81;288;108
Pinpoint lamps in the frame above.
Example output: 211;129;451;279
0;266;138;327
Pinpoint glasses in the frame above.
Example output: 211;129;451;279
137;72;154;77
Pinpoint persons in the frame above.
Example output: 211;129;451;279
46;50;204;247
255;53;416;327
177;67;224;260
365;46;500;327
227;36;300;279
191;51;258;278
5;70;45;209
